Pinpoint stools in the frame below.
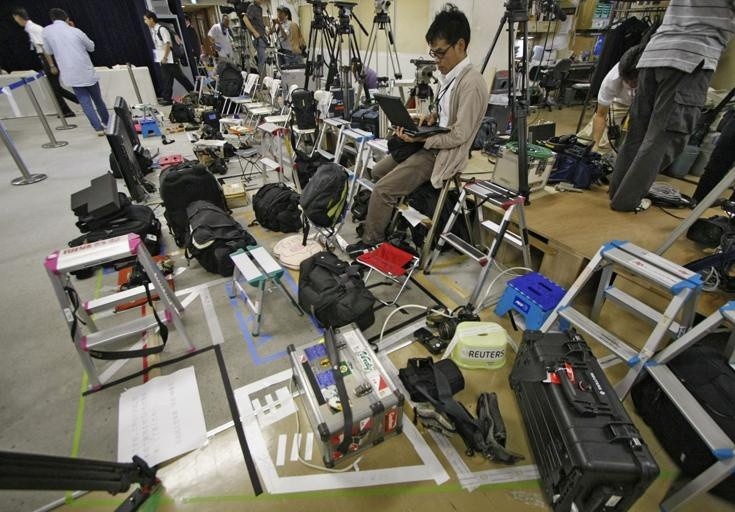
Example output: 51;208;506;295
450;321;507;371
356;242;421;308
227;243;304;336
140;119;162;138
494;270;570;333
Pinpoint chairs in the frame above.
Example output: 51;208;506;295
221;70;333;161
529;58;572;112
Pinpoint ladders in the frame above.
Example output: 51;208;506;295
539;239;706;402
388;172;473;272
612;301;735;512
424;178;532;311
45;233;196;391
318;118;388;204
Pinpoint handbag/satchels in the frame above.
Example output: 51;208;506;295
386;132;426;165
297;251;377;335
179;200;256;276
291;89;317;129
627;339;735;504
250;180;303;234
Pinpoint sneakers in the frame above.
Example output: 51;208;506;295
636;197;652;212
346;241;379;256
97;129;107;137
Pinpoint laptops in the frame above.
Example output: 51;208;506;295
373;93;451;138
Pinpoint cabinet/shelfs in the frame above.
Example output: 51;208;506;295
231;18;250;60
576;0;632;31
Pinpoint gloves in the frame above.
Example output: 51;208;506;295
49;64;58;75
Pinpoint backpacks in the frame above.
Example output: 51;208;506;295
404;179;462;252
168;101;195;124
68;204;162;268
168;29;185;59
349;106;379;139
297;161;350;230
291;150;320;190
154;159;231;243
217;62;244;97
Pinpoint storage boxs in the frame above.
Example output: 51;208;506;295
491;141;559;195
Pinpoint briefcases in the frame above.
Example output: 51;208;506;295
507;325;662;512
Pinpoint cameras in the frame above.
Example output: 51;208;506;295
439;303;480;340
608;125;621;140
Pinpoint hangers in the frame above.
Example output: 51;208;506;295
607;5;666;35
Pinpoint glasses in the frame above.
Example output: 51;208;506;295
427;40;457;58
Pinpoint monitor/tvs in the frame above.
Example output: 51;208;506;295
106;110;156;203
114;96;153;168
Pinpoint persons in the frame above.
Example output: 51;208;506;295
143;10;194;106
207;15;235;59
207;62;232;89
40;8;109;137
242;0;306;85
351;57;378;97
185;13;203;64
346;2;489;258
589;42;649;152
12;5;80;117
609;0;735;212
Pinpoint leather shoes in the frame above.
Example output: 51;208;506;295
158;99;173;106
57;111;75;118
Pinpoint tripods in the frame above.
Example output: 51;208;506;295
479;10;536;205
240;24;258;75
325;19;372;104
304;14;340;91
1;450;161;512
654;87;735;258
350;14;406;114
